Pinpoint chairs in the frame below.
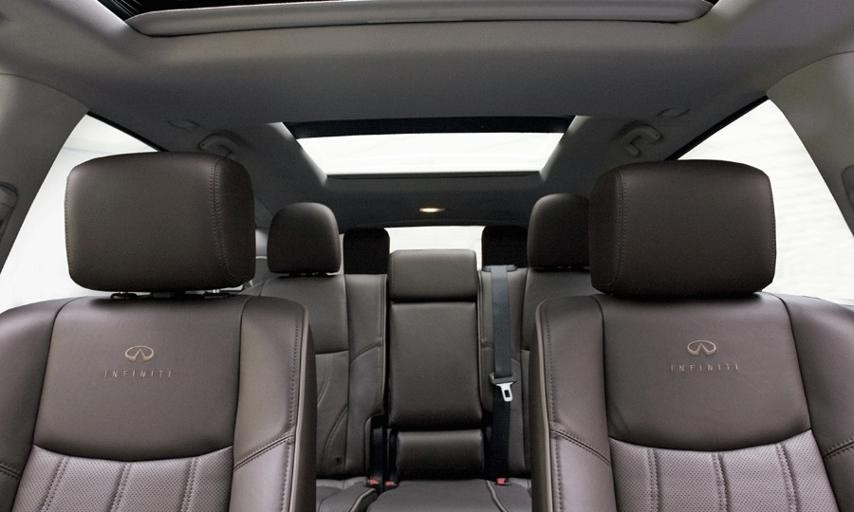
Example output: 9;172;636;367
1;153;318;512
526;153;853;511
236;191;587;512
